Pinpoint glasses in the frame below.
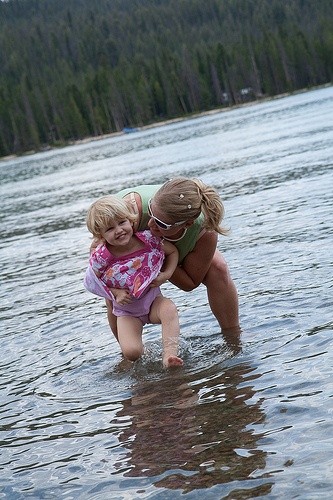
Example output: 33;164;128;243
147;198;188;230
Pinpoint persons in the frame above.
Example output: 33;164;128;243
221;87;255;107
84;195;184;368
90;176;242;347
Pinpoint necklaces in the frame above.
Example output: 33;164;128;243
160;228;188;246
